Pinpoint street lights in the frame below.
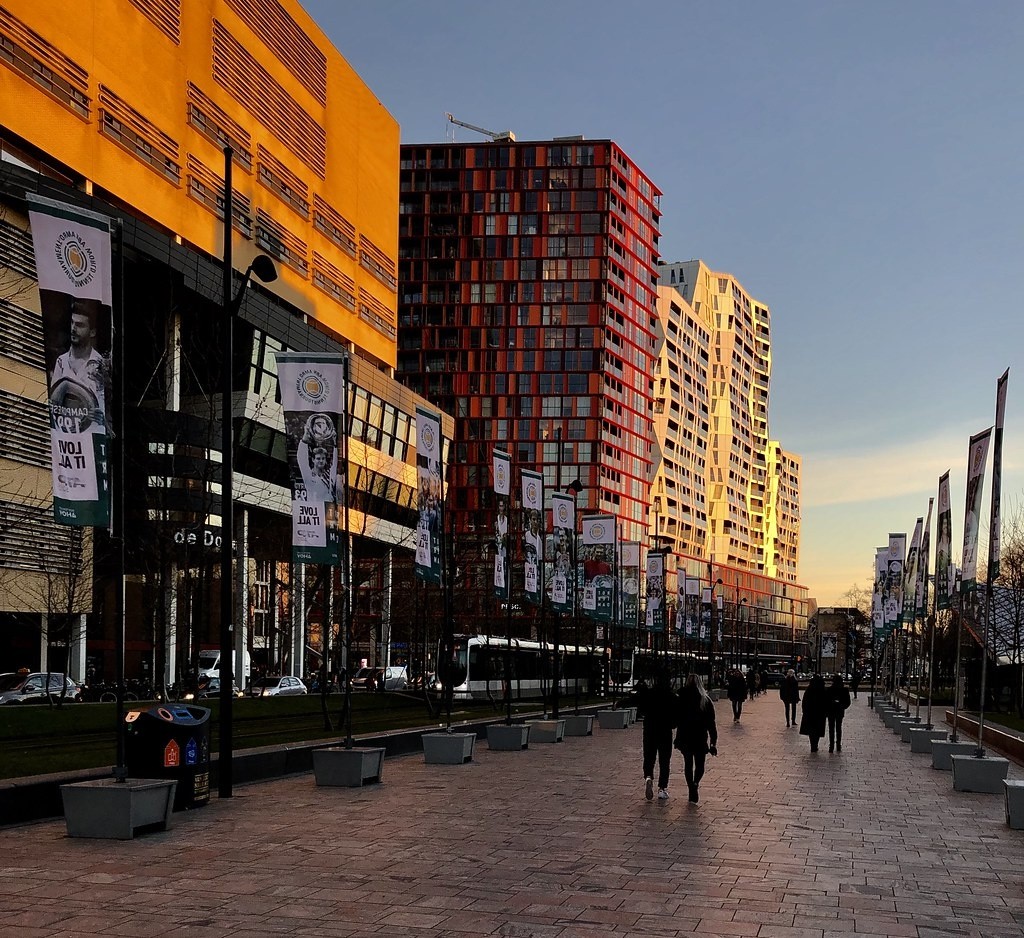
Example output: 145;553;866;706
553;426;583;635
707;554;723;697
735;577;747;669
654;510;672;666
219;140;277;799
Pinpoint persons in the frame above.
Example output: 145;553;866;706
585;546;610;584
678;587;684;614
645;581;663;609
938;518;949;559
524;509;542;561
495;500;507;557
291;424;338;502
49;302;106;425
726;668;851;753
417;475;441;528
611;667;717;804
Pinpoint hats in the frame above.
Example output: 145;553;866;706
787;669;796;674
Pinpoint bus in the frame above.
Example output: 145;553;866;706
607;644;726;695
422;631;613;703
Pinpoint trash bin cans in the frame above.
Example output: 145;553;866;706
130;704;214;809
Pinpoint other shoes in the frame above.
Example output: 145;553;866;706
787;724;789;727
829;747;834;753
837;746;841;751
735;713;739;719
734;720;739;723
657;789;669;799
792;723;797;725
688;783;699;803
811;745;818;752
645;776;653;801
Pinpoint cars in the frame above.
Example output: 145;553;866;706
238;675;309;699
158;683;240;702
756;670;919;682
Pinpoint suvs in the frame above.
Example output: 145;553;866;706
1;671;81;704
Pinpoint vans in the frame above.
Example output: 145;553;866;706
349;666;409;691
188;648;251;684
